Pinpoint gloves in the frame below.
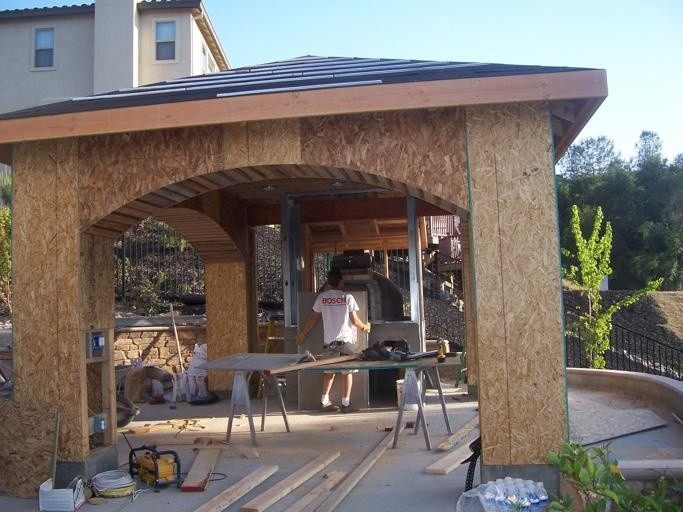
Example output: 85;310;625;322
362;321;371;334
295;330;307;346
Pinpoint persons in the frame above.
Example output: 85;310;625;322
292;267;371;414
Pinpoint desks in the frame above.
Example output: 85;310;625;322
193;351;465;449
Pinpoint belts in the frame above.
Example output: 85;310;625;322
323;340;345;349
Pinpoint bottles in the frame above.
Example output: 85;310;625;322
483;476;548;512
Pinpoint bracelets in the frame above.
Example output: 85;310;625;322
361;324;365;332
301;330;306;337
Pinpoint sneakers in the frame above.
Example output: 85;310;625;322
340;402;359;413
319;401;339;412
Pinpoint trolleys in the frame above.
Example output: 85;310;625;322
114;366;140;427
